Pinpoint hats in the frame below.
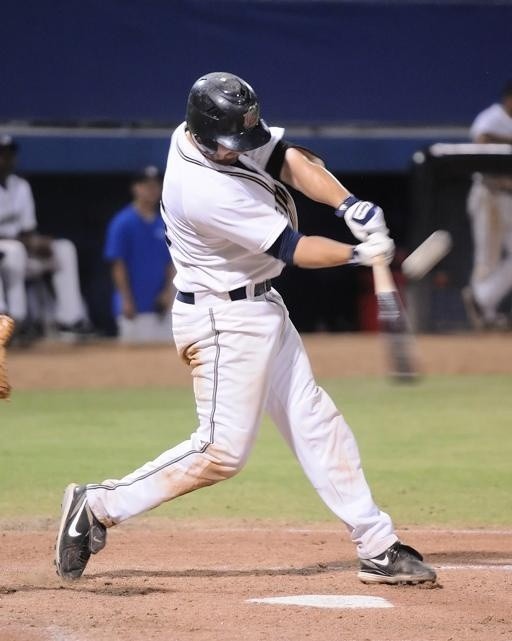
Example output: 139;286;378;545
131;165;162;183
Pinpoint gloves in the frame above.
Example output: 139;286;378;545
334;194;392;241
348;231;396;268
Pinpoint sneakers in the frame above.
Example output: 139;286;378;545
53;482;106;584
358;540;436;585
462;285;509;329
57;317;94;336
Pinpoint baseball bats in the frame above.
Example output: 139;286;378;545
372;250;422;383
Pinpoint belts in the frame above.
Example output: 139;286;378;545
176;279;272;304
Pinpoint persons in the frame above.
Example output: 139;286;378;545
101;164;175;343
1;135;95;347
461;84;510;328
53;72;442;591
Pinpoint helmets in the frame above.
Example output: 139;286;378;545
185;71;271;155
